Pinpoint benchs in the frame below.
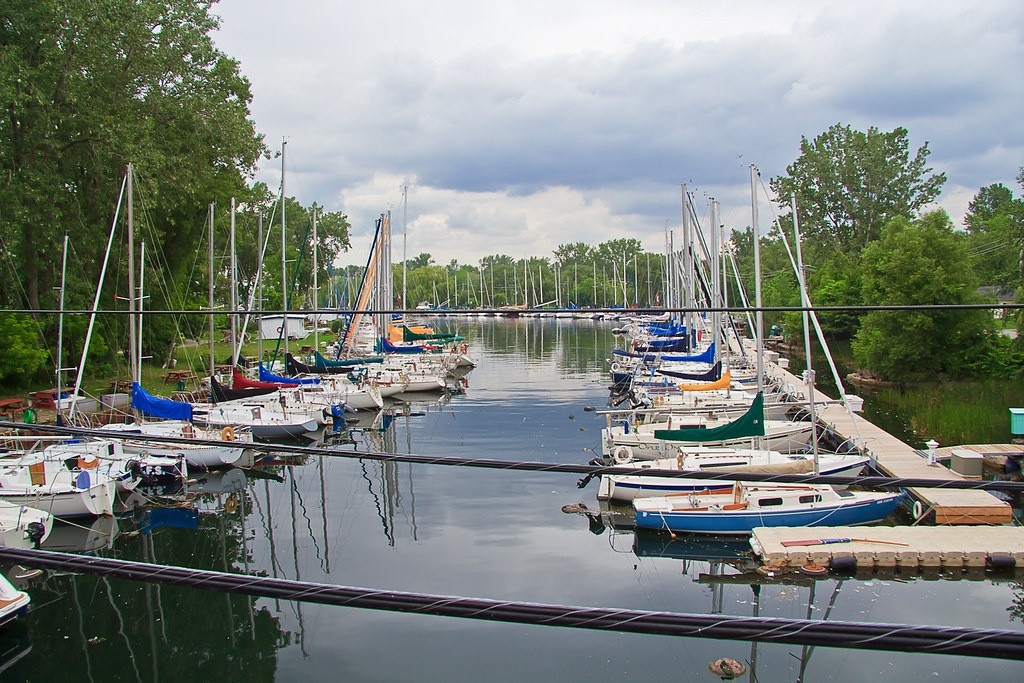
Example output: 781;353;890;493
11;406;32;421
301;344;312;355
249;361;258;364
179;376;198;384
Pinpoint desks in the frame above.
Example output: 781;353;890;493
245;356;259;367
214;365;231;374
33;386;78;412
0;397;33;428
163;369;199;387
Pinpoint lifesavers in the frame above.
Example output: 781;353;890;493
222;426;236;441
912;501;923;519
655;396;665;406
611;362;621;372
614;444;633;463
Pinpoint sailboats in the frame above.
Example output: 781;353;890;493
0;132;906;657
